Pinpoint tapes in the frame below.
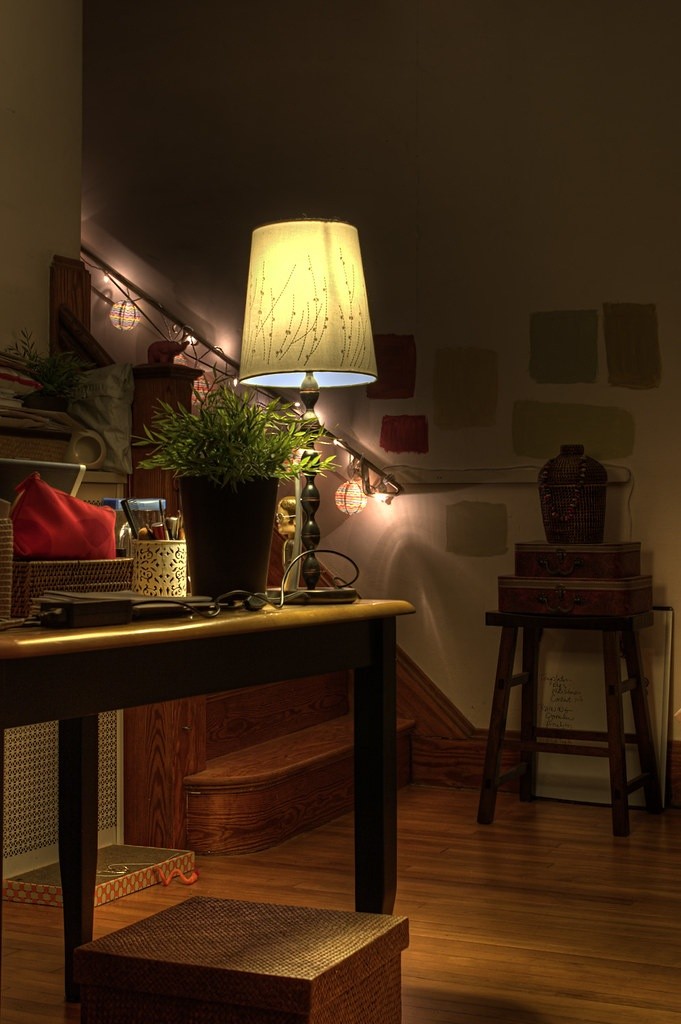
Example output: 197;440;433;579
63;429;107;470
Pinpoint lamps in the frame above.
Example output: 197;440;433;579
237;218;379;603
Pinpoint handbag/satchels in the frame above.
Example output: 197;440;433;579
7;471;117;562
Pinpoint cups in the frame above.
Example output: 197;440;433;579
132;540;188;599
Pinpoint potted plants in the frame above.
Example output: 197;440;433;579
131;360;340;614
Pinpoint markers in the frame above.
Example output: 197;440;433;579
150;523;164;540
165;517;178;540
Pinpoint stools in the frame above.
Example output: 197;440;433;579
475;611;662;836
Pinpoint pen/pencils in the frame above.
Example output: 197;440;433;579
119;497;185;542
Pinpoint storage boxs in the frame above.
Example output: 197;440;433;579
73;895;410;1024
497;573;653;615
514;539;642;579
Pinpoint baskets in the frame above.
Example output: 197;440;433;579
10;556;135;618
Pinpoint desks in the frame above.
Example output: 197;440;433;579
0;600;415;1002
